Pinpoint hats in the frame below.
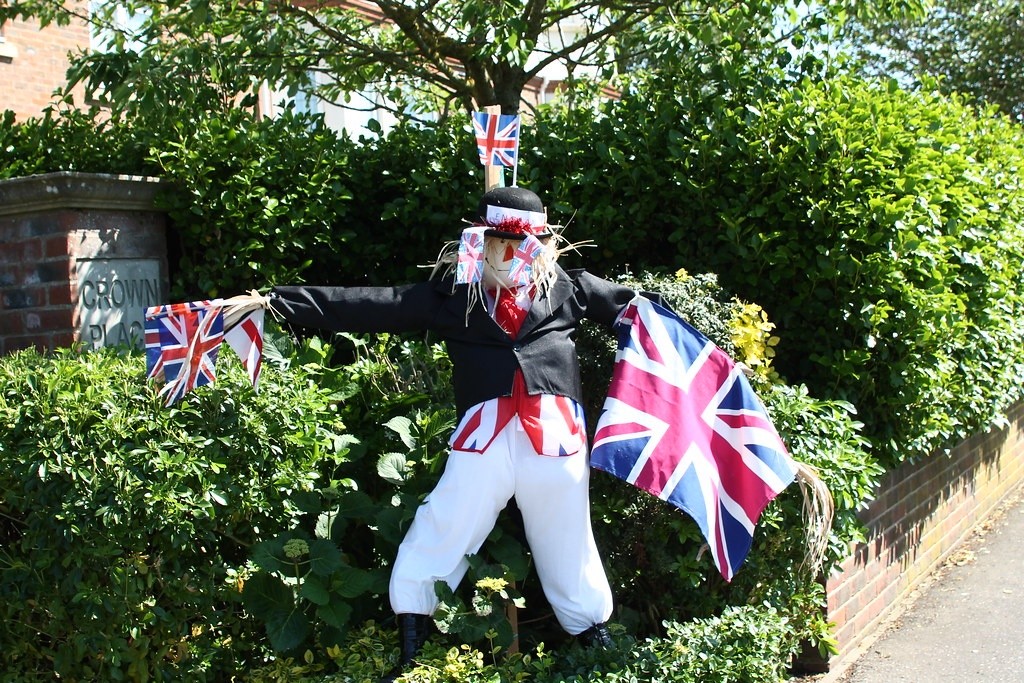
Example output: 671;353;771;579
461;185;556;238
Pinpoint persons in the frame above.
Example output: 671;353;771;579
271;187;664;683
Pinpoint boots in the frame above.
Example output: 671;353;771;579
577;624;620;654
381;613;430;682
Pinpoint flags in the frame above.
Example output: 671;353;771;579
145;299;264;408
456;226;496;284
590;295;801;585
507;235;544;286
472;111;521;166
